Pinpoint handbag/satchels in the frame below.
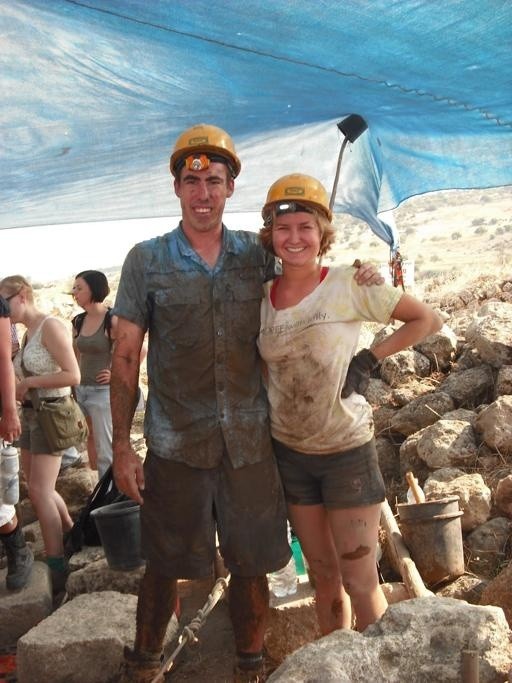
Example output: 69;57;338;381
79;489;132;547
37;395;90;452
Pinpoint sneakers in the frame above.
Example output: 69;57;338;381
123;645;166;683
47;556;72;611
232;655;265;683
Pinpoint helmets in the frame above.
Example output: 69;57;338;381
169;123;241;180
261;174;332;224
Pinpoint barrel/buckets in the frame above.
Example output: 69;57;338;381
398;511;465;582
393;495;464;512
90;500;143;572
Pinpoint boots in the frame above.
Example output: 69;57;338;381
0;521;35;590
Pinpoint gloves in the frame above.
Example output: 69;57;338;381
341;348;380;399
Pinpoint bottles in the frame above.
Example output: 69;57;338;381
0;443;19;506
406;478;425;506
265;519;298;598
290;536;306;576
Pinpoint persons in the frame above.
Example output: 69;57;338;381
110;124;294;683
256;173;442;635
0;275;81;612
0;293;33;590
70;270;114;496
